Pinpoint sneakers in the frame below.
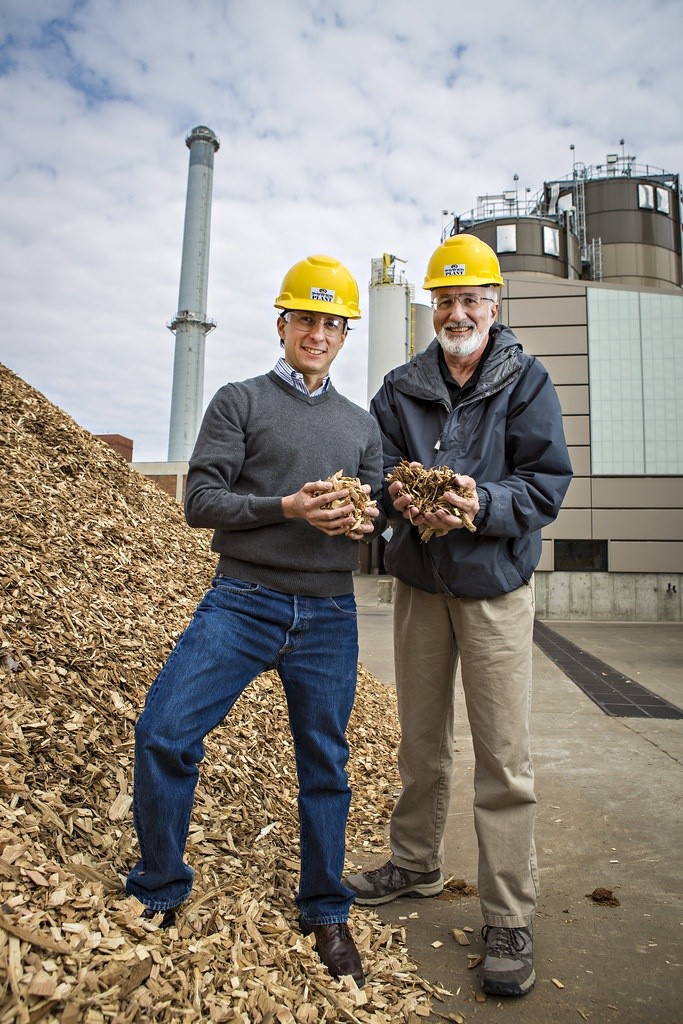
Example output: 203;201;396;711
481;921;536;996
343;862;446;905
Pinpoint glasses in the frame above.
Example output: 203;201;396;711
280;311;348;338
429;292;495;311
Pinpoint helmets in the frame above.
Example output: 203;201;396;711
274;255;361;321
422;233;505;290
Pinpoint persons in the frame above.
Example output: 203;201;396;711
127;254;383;988
344;234;574;994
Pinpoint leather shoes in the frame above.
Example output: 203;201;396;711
112;898;179;931
299;913;365;988
